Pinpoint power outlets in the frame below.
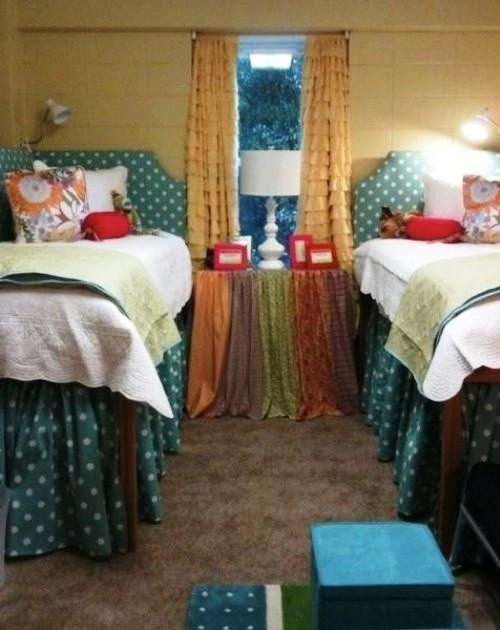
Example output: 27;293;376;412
21;135;31;148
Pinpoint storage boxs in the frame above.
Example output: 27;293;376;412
310;522;456;629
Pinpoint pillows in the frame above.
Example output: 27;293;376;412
407;217;464;241
463;175;500;244
354;152;426;247
81;213;131;242
33;160;128;212
4;165;90;242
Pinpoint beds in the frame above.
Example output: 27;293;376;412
0;144;188;558
353;149;500;554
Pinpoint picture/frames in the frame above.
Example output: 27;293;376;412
213;243;247;270
288;235;312;269
306;243;338;269
232;236;252;263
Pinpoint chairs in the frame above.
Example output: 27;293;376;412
447;460;500;574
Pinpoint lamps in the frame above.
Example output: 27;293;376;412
19;96;71;153
461;107;500;144
240;150;302;271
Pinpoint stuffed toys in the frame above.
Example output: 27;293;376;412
379;206;423;238
111;190;160;234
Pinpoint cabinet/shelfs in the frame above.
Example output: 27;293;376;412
188;585;466;630
185;268;359;423
